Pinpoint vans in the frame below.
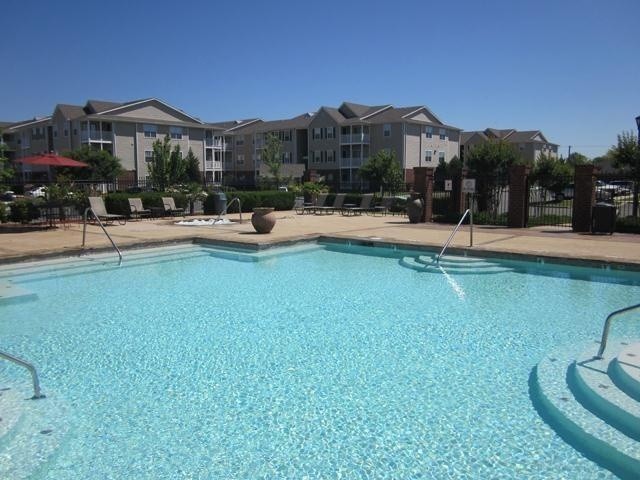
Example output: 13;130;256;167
0;190;18;224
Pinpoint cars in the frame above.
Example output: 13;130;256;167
547;179;633;204
169;184;225;194
279;187;288;192
24;185;75;200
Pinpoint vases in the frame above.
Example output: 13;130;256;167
250;205;276;233
407;192;424;224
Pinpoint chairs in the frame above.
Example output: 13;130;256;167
128;198;155;221
162;196;186;222
292;193;409;218
87;195;127;228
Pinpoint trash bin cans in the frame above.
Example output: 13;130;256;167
591;203;618;235
212;192;227;215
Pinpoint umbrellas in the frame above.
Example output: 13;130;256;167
10;150;88;181
